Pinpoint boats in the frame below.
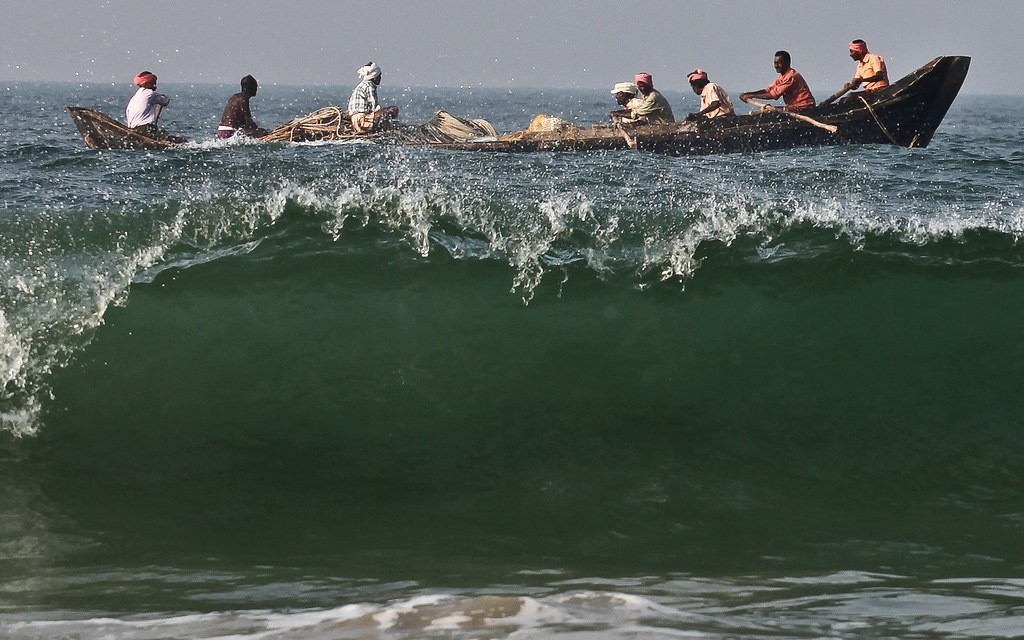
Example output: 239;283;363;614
62;51;974;148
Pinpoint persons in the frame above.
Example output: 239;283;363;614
123;69;172;140
607;73;674;127
848;36;889;94
739;51;816;119
217;73;270;142
350;60;400;139
683;69;734;125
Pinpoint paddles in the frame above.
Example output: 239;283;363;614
746;96;838;133
818;86;853;104
611;115;634;149
155;106;164;121
